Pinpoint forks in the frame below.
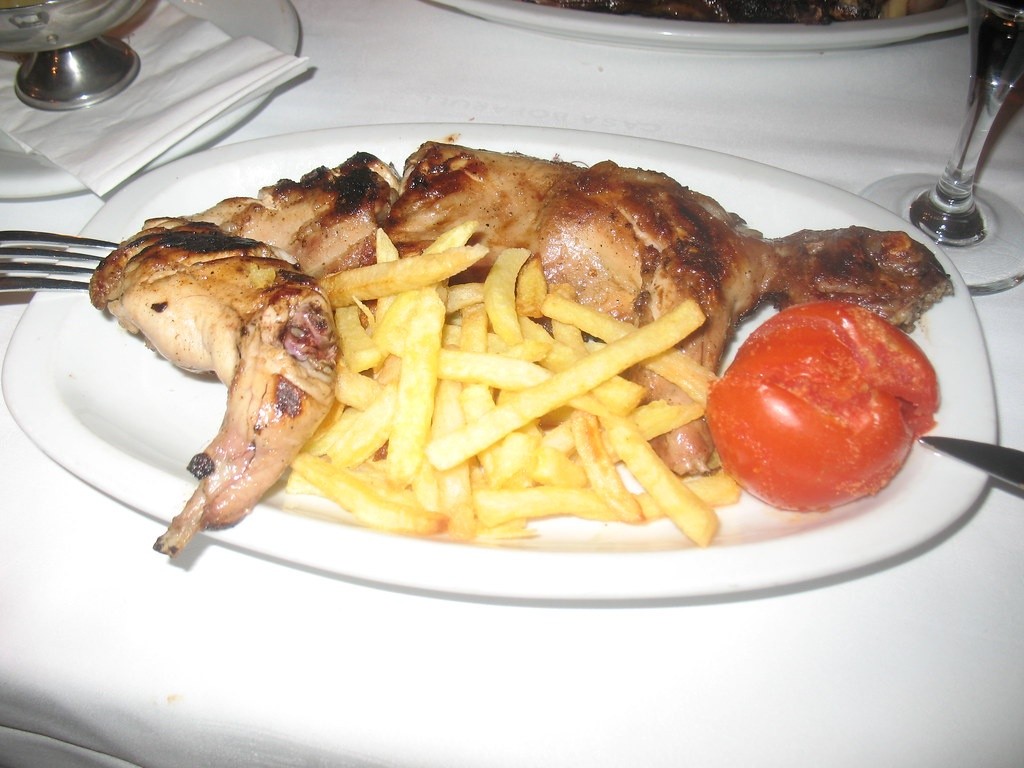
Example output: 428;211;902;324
0;230;120;293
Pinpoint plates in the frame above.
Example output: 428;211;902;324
0;0;301;199
0;0;148;111
4;122;1001;601
431;0;972;50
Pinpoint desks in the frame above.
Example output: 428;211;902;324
0;0;1022;767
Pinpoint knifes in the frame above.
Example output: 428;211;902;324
917;436;1024;491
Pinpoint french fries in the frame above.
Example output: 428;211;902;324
287;217;743;548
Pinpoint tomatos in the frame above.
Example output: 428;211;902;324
703;300;939;511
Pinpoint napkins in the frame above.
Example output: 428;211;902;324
0;0;310;202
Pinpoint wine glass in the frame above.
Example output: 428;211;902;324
854;0;1024;295
